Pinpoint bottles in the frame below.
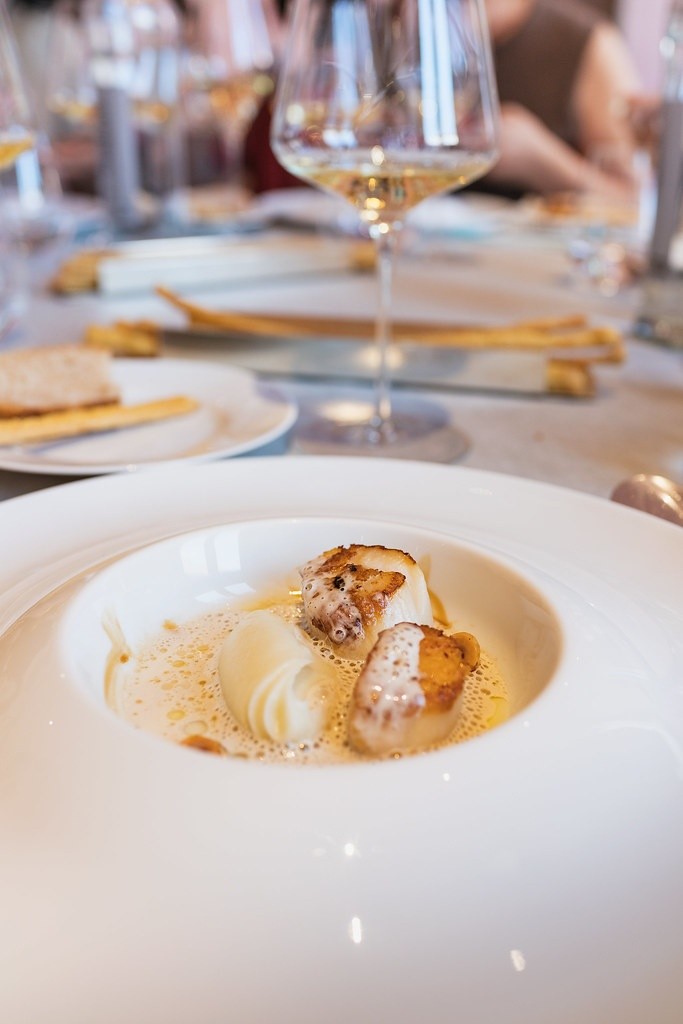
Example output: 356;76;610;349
637;0;683;274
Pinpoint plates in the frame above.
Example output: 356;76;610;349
0;454;683;1023
0;358;299;474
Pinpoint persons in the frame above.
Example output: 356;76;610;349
242;1;643;219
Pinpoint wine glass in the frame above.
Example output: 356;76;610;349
0;2;183;346
270;0;501;466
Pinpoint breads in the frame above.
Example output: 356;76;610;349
0;344;119;417
